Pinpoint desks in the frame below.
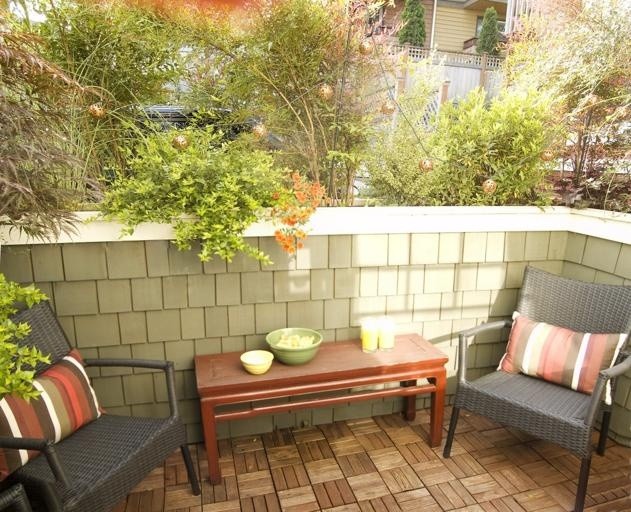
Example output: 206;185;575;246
193;329;451;496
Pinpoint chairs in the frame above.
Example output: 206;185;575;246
443;260;631;510
0;298;204;512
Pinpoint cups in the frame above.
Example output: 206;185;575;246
360;317;379;353
377;319;398;353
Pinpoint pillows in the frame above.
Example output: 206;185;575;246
497;311;627;408
1;344;104;488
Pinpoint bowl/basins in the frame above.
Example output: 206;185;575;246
266;327;324;366
240;349;276;375
